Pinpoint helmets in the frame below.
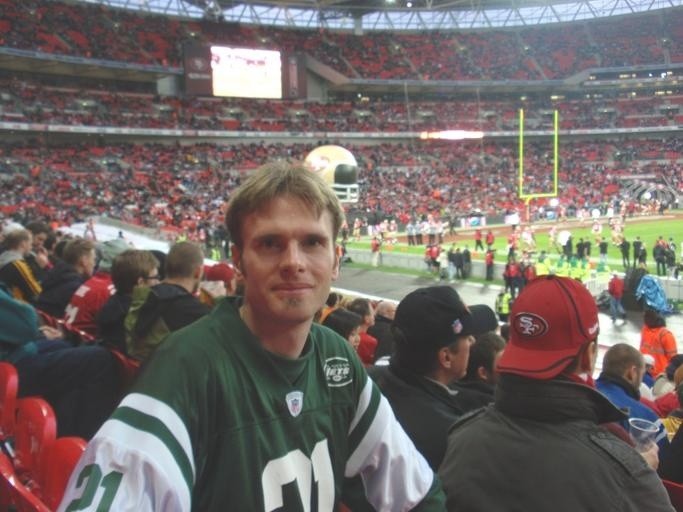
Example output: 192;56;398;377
303;146;360;202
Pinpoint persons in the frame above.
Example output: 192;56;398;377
55;157;449;512
0;0;683;487
437;275;674;511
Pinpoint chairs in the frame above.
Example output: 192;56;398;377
0;0;682;232
660;478;682;512
1;308;157;511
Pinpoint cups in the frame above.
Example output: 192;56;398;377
628;418;659;454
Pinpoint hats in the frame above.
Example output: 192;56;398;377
496;274;598;378
207;264;236;280
392;286;498;347
